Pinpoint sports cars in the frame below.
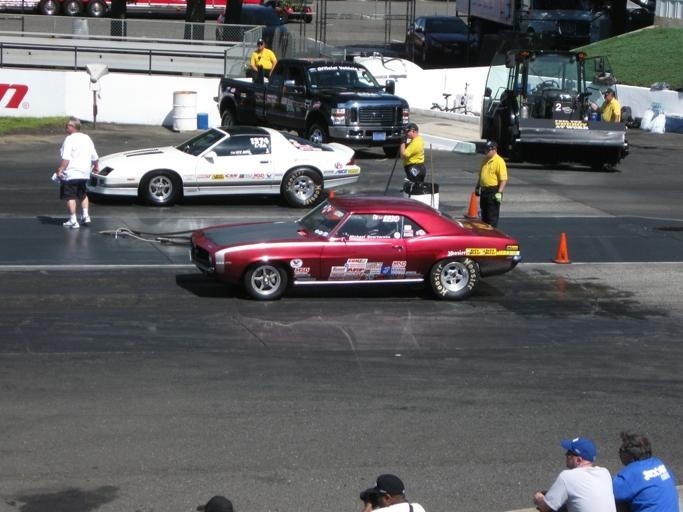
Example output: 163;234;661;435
189;193;521;299
84;127;361;208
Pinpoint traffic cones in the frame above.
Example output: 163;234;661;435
462;191;480;219
553;230;570;265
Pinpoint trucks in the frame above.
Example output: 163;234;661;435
456;1;591;47
0;0;275;17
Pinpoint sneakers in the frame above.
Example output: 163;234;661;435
62;220;80;229
79;216;92;225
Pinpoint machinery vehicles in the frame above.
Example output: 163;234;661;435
481;47;628;165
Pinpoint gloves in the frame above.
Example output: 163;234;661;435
400;136;407;143
475;187;480;197
492;192;503;203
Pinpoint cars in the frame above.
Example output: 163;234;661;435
269;0;313;22
216;5;287;47
407;17;481;63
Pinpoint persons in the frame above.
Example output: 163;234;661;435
55;116;99;229
359;487;379;512
587;87;621;123
250;37;277;81
361;215;380;237
474;141;508;228
399;123;426;182
533;436;617;512
611;433;679;512
370;474;427;512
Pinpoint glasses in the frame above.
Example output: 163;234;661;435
605;95;610;98
65;123;75;128
257;42;264;45
404;130;414;134
484;147;496;151
567;449;578;456
619;446;627;453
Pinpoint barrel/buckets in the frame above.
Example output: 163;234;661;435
172;92;197;131
197;113;208;129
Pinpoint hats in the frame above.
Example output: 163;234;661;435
602;88;615;94
403;123;418;132
484;140;498;148
560;435;597;462
367;474;405;496
257;38;265;43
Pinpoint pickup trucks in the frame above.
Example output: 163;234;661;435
215;58;408;158
